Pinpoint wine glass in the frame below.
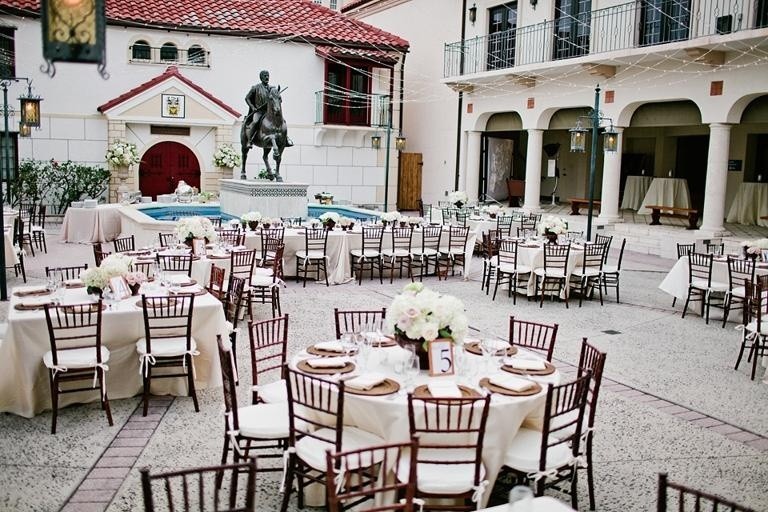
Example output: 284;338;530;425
449;331;501;378
339;320;422;379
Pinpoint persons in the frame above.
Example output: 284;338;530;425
244;69;295;150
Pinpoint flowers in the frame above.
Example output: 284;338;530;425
449;190;467;205
101;139;145;167
213;143;242;168
199;191;216;201
740;240;761;261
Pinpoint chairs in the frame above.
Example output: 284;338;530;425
672;243;768;380
505;176;524;207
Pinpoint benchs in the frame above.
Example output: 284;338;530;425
567;199;601;215
646;206;700;230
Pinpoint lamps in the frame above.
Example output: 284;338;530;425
468;3;476;26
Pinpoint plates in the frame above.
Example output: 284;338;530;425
339;374;400;398
295;356;357;376
182;217;466;235
442;200;529;228
124;235;247;265
306;323;400;356
70;192;214;209
477;374;543;396
501;230;590;250
11;270;208;312
500;353;560;377
462;338;519;358
412;380;484;407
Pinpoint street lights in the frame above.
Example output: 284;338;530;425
572;83;619;243
368;121;406;211
0;74;43;205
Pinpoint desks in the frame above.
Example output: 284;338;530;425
625;176;687;222
740;182;768;226
